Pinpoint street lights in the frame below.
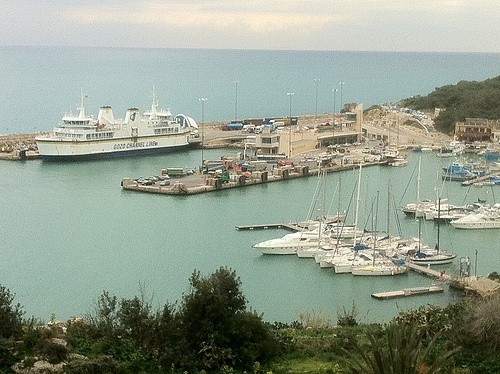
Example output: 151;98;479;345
232;80;239;122
286;92;294;162
314;78;321;124
338;80;347;120
198;97;207;168
332;88;340;147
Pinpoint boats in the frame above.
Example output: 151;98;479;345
34;77;202;159
371;286;444;299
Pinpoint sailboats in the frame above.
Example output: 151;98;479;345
251;134;499;274
